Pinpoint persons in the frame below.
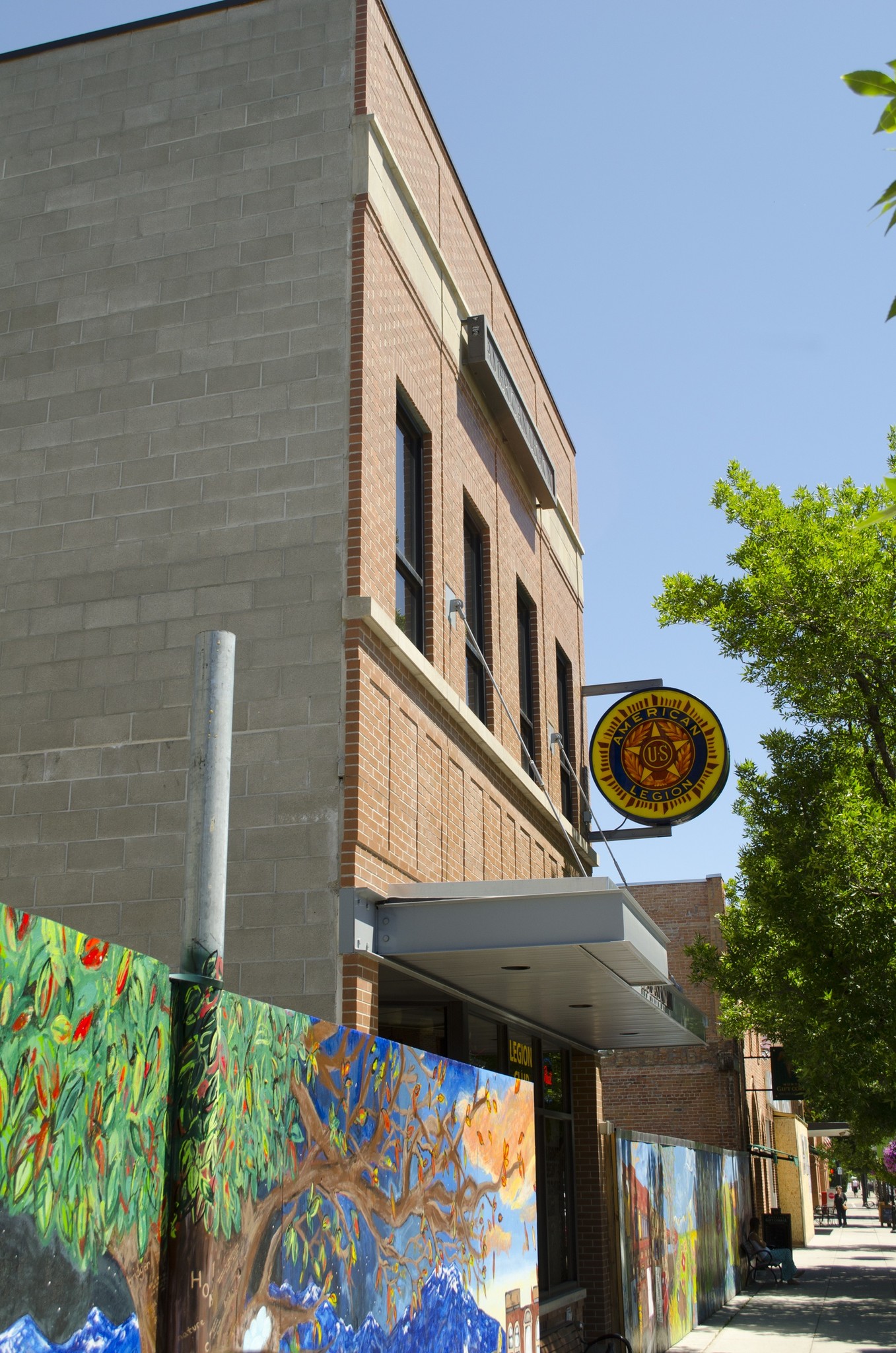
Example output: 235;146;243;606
748;1217;805;1285
852;1179;859;1199
833;1186;848;1228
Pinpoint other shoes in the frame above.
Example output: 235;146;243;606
788;1279;800;1285
794;1270;805;1278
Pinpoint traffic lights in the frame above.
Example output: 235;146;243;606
830;1168;835;1175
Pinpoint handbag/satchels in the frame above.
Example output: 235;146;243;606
842;1204;848;1210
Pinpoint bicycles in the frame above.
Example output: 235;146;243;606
864;1201;879;1210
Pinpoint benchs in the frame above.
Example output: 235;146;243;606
861;1195;875;1209
741;1241;783;1289
536;1321;632;1353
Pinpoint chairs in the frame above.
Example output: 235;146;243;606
813;1205;839;1228
